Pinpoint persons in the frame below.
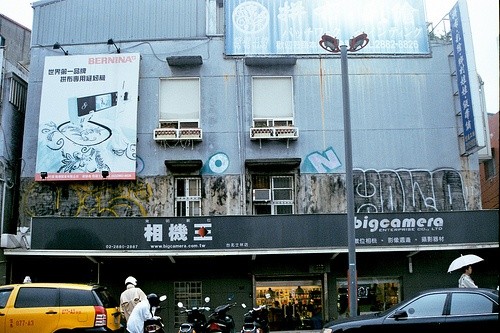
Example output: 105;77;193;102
126;293;161;333
120;277;148;325
458;266;478;288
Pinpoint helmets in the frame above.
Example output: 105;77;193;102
124;276;137;285
148;293;159;307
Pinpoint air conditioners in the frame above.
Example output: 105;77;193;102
252;189;271;202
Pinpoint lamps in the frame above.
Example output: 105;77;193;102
54;42;68;56
102;171;108;179
107;39;120;53
41;172;48;179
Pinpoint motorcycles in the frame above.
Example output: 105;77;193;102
241;292;273;333
205;293;237;333
143;294;168;333
178;297;213;333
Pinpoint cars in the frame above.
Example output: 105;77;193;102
320;287;500;333
0;276;122;333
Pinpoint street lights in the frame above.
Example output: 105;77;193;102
319;33;370;316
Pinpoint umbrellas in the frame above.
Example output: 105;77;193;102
447;253;484;273
